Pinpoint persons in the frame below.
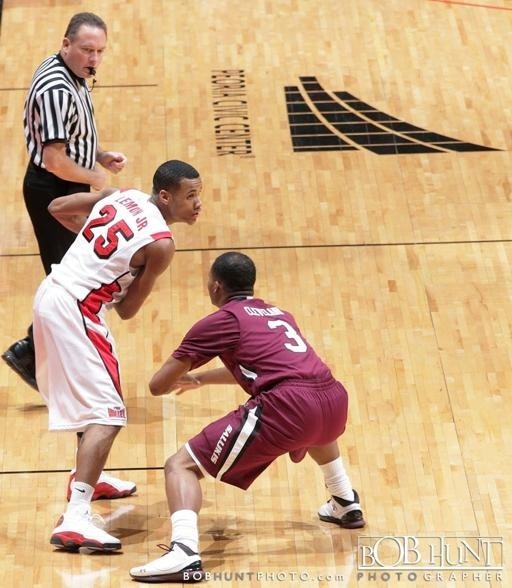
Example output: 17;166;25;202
33;159;203;552
129;251;365;583
1;12;127;394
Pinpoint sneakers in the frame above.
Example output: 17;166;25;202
48;512;121;550
67;468;135;501
130;542;205;581
2;338;38;390
318;489;364;528
